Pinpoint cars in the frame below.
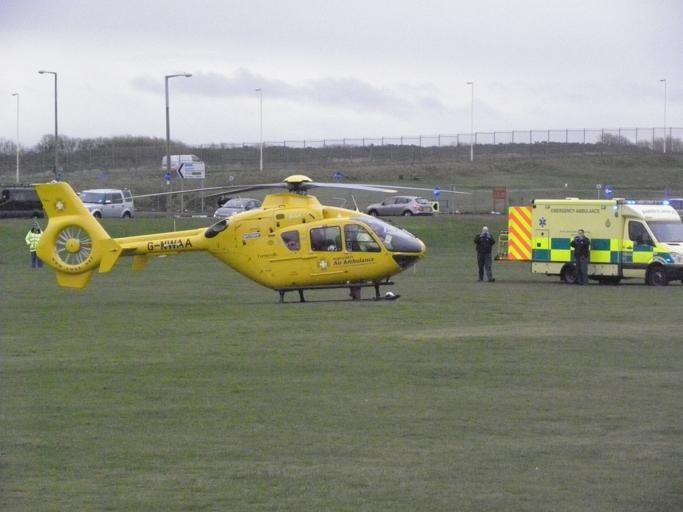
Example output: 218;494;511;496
213;197;262;222
365;195;434;217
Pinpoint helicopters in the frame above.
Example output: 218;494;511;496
29;173;474;303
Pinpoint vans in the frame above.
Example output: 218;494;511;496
0;186;44;219
77;188;135;220
162;155;201;172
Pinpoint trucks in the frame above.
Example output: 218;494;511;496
532;198;683;287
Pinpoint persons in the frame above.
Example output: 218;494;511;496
355;225;384;252
570;230;591;285
473;226;496;281
24;222;44;269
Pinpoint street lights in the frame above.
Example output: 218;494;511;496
11;92;19;185
660;79;667;153
255;88;263;173
466;81;473;161
38;70;60;181
164;73;192;216
